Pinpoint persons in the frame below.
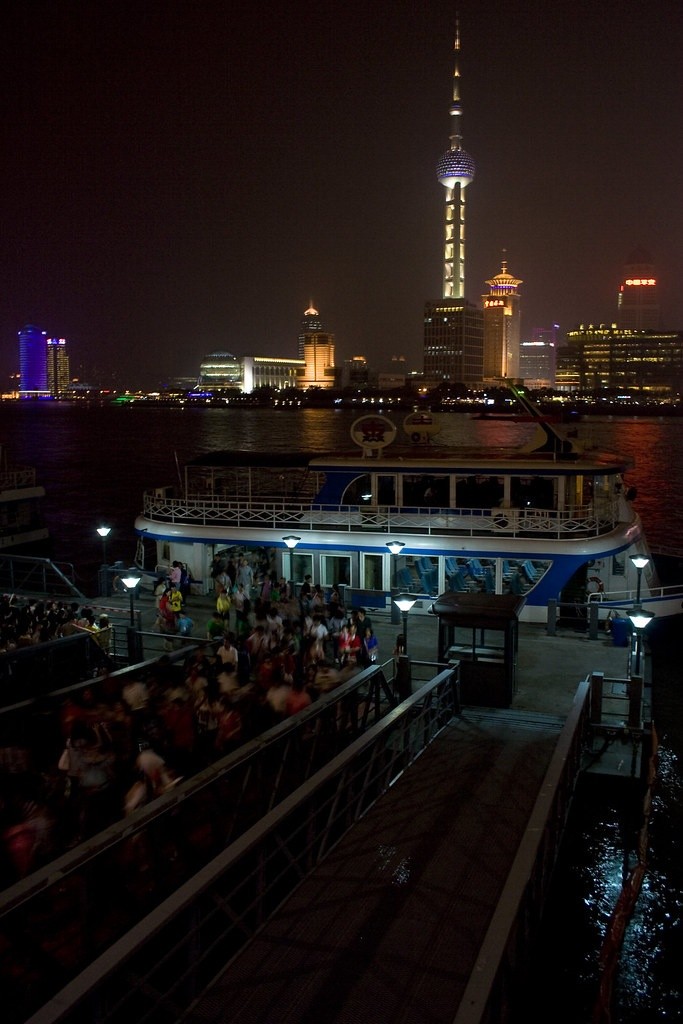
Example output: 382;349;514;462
0;552;379;884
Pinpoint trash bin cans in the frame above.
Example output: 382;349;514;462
612;618;629;647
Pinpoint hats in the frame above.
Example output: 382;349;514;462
138;750;165;770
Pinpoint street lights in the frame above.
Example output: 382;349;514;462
391;587;417;701
97;523;112;596
281;534;302;601
385;541;407;625
628;551;650;650
625;607;654;730
119;572;139;668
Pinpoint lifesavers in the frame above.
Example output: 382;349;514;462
583;576;605;598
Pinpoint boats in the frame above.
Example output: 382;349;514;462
133;374;663;635
411;410;434;425
